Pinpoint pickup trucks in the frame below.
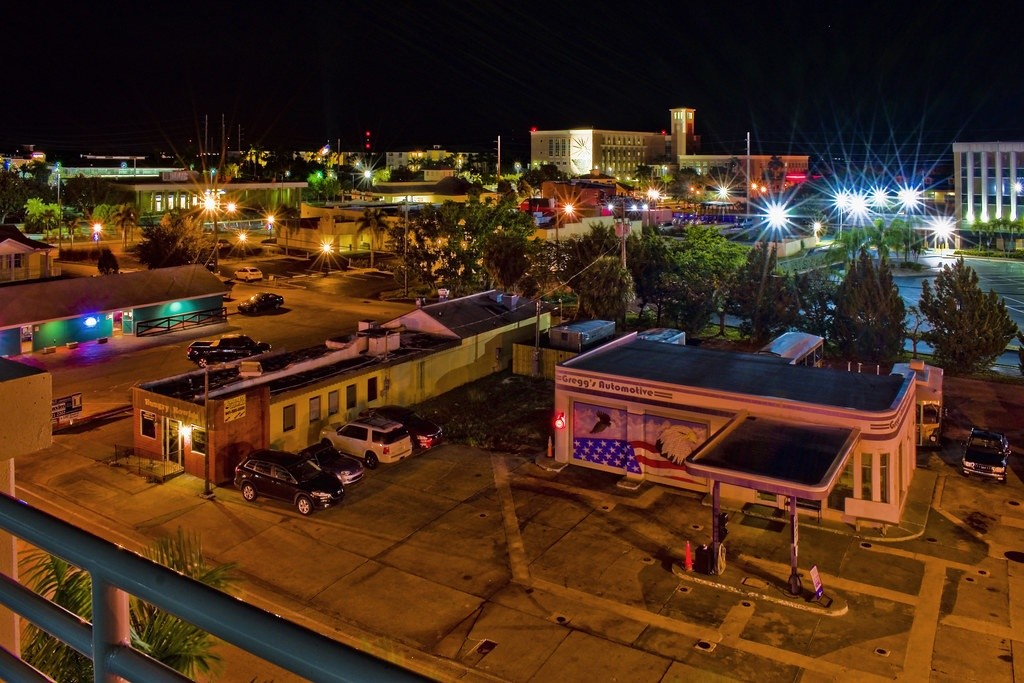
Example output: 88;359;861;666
186;334;272;368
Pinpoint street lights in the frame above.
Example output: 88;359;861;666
281;170;291;207
93;223;102;247
323;244;332;276
404;195;412;296
838;184;950;267
650;189;660;224
240;234;246;262
197;361;263;498
516;163;521;174
205;169;236;275
768;201;786;272
352;161;371;191
267;215;273;239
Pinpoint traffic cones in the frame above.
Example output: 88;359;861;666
681;540;695;573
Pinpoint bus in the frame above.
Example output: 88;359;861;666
754;331;824;368
888;359;947;450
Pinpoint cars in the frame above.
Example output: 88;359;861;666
604;195;650;216
960;428;1011;482
516;198;558;228
234;266;284;315
658;223;687;235
234;405;443;514
